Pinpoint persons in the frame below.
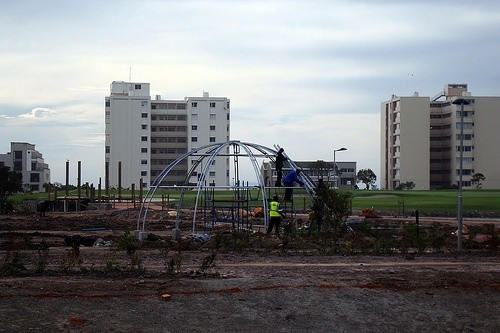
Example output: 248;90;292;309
316;178;327;195
266;196;282;235
274;148;287;187
312;199;321;229
282;168;304;202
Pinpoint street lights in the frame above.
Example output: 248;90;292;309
333;148;347;189
452;98;469;260
